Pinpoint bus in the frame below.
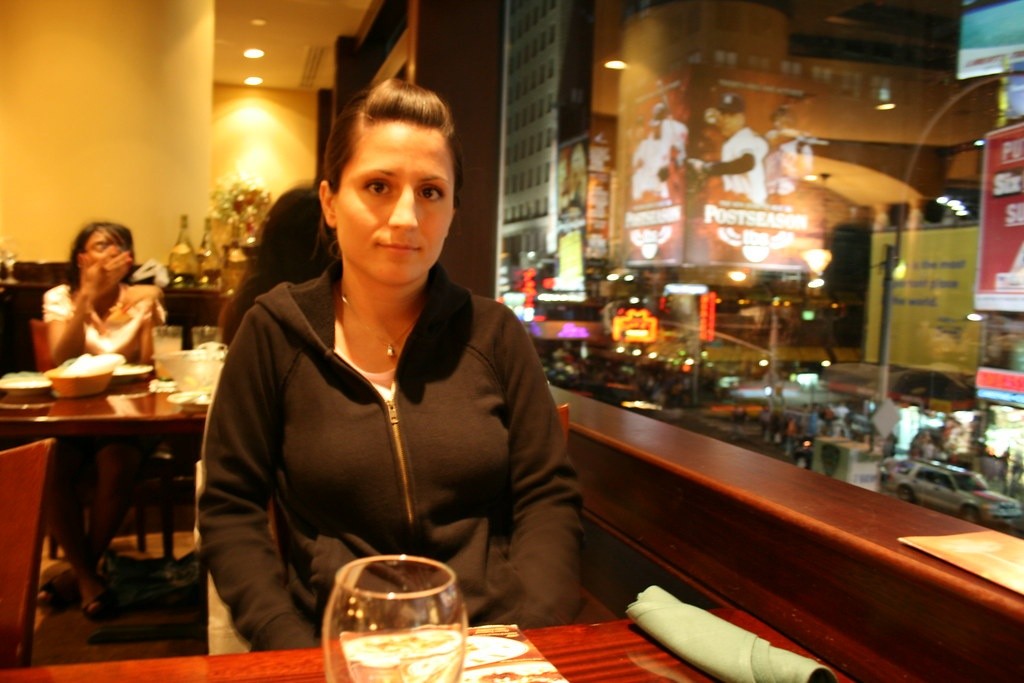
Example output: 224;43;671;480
584;350;717;412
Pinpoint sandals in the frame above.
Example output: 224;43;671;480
36;573;72;609
81;574;113;619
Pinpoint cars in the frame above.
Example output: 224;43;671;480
544;361;587;391
795;445;889;484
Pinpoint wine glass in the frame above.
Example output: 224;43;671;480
0;239;20;283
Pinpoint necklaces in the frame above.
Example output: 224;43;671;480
337;285;423;357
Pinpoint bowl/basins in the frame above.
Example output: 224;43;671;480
45;367;113;397
154;351;221;387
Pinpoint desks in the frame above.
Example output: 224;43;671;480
0;609;855;683
0;379;210;567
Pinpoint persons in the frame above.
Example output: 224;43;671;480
196;78;584;653
216;186;342;349
663;380;1024;497
632;92;804;206
43;222;167;621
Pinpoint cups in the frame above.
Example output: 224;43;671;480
323;557;467;683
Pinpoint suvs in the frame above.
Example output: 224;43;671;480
888;459;1022;527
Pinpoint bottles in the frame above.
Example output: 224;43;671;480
168;213;197;285
198;218;220;284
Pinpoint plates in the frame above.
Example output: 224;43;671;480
461;635;527;669
112;364;153;380
0;378;50;395
169;392;211;411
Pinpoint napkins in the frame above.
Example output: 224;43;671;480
626;582;837;683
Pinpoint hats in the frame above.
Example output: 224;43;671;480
650;102;667;127
717;94;744;114
